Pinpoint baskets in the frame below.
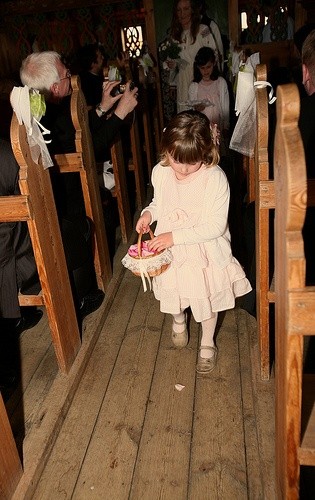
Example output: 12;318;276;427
127;227;168;278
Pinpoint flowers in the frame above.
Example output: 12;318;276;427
210;118;222;144
158;38;186;63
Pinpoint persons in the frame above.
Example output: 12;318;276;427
188;47;230;140
1;43;147;330
267;23;315;289
162;0;224;114
136;111;252;373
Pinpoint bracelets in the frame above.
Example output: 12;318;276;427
96;104;106;115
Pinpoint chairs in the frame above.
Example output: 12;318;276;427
229;45;315;500
0;55;160;500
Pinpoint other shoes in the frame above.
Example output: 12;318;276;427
15;309;43;338
171;311;189;349
194;345;218;375
76;288;105;317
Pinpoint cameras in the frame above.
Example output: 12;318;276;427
118;82;134;94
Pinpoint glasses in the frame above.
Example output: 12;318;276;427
59;68;72;81
